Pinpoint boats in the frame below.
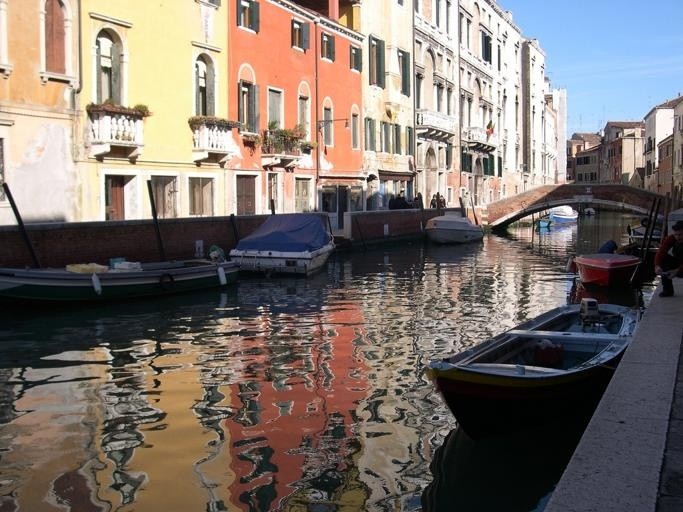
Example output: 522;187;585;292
584;207;596;215
546;205;579;225
536;219;554;227
5;294;227;358
422;296;643;441
564;279;646;311
420;211;486;246
564;218;664;291
535;227;551;235
0;241;245;307
229;210;338;280
419;408;591;512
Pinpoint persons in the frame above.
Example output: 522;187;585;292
653;220;682;297
388;190;446;210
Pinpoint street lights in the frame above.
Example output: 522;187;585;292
314;117;351;210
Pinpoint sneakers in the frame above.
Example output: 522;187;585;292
659;291;674;297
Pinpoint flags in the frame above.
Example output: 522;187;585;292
486;119;493;136
